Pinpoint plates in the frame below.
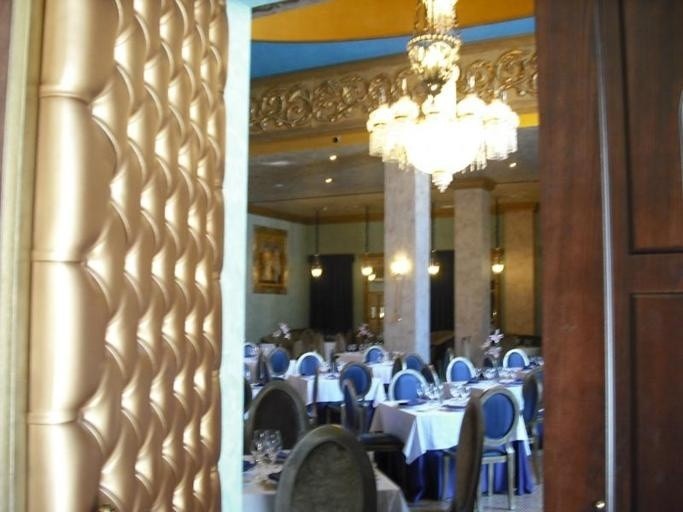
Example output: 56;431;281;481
252;476;277;495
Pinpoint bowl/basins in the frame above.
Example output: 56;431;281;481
518;370;531;380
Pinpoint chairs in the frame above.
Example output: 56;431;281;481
244;326;543;512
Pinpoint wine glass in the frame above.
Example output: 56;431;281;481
416;381;471;408
475;366;522;381
318;361;345;379
250;428;281;479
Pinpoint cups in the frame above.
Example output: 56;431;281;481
377;351;405;363
346;343;367;354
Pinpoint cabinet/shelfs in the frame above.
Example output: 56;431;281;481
360;252;384;329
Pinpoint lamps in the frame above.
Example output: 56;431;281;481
427;201;441;275
310;207;323;278
360;206;373;276
365;0;521;195
491;192;504;274
